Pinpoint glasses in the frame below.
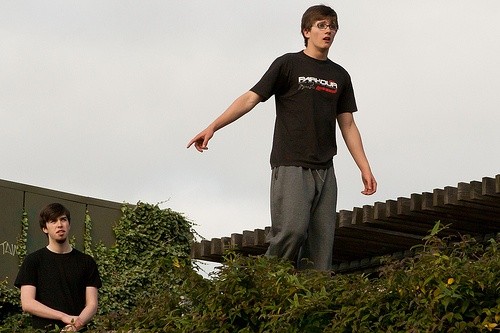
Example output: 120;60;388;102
307;23;337;31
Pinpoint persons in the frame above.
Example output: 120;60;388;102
187;5;377;276
14;203;103;333
